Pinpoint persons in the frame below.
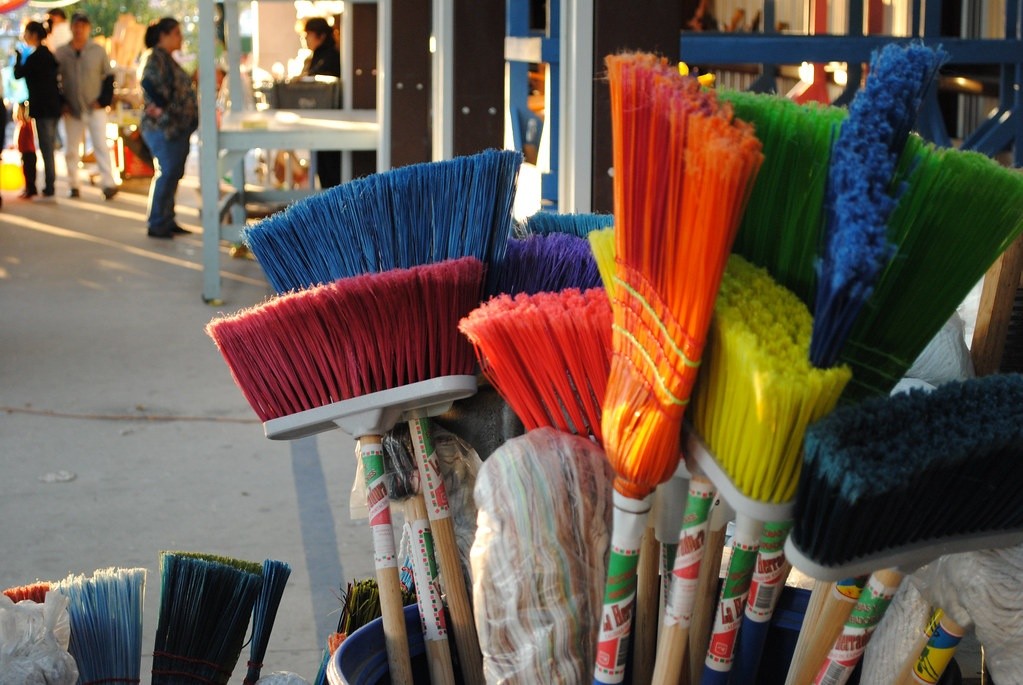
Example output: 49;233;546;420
13;18;64;198
52;12;122;201
16;102;37;199
46;9;73;52
301;18;342;188
137;17;198;238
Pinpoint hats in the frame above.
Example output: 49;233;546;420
71;12;89;23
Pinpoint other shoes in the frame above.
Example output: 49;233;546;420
104;187;119;199
71;189;79;197
148;220;192;239
